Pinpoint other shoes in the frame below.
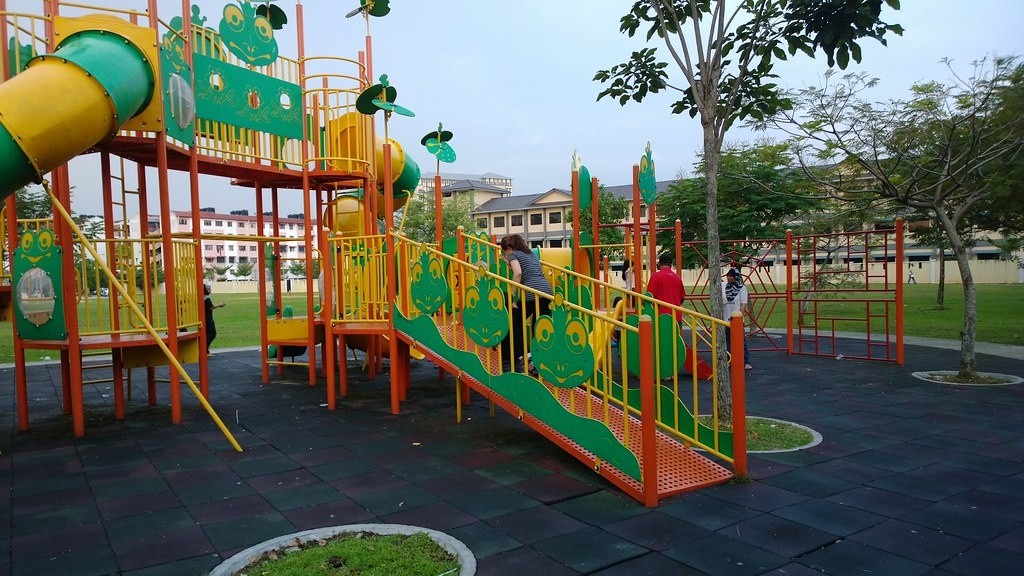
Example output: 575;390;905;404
745;362;752;370
503;360;510;371
531;368;538;375
515;360;523;372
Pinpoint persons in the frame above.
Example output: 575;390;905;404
622;259;636;307
646;252;686;382
908;264;917;284
720;268;753;370
501;292;523;361
611;296;624;357
501;234;554;377
203;283;226;356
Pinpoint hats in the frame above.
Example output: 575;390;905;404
659;252;672;263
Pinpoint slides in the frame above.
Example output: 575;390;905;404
267;305;321;358
0;35;155;200
323;124;593;299
